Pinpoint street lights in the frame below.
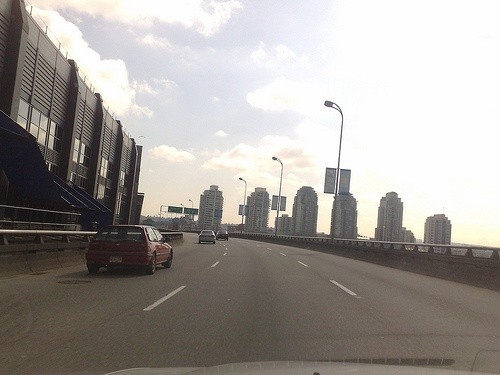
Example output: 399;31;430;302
238;177;247;238
180;187;221;233
324;100;343;249
272;157;283;241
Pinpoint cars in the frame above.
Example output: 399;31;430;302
86;224;174;274
198;230;216;244
216;230;229;240
